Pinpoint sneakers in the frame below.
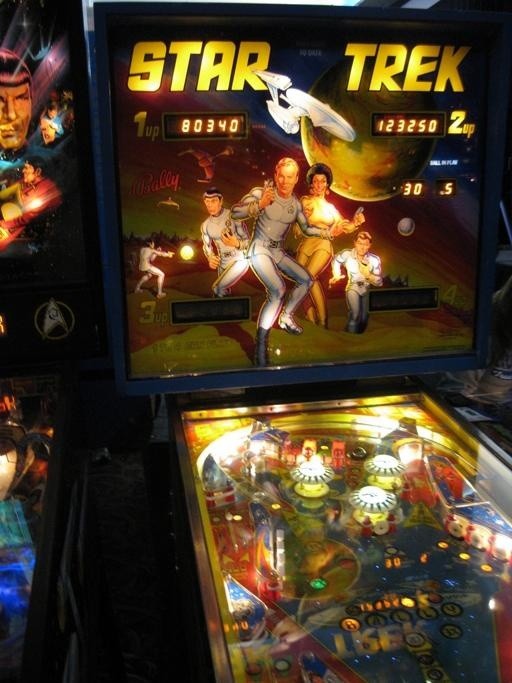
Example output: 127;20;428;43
135;289;143;293
157;293;166;298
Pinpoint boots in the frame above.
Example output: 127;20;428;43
278;283;307;334
256;327;269;366
